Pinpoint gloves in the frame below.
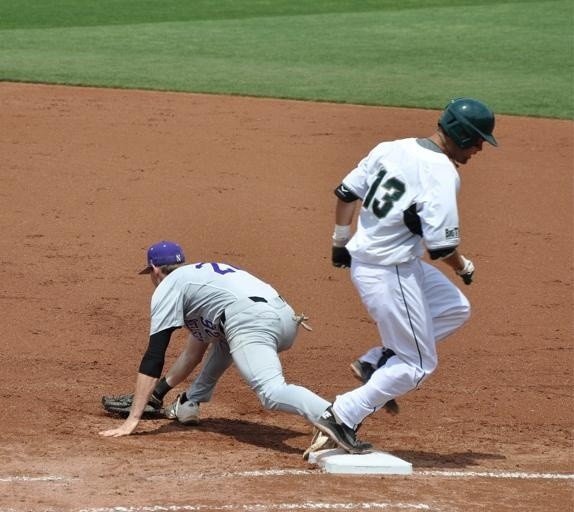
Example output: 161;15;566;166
454;256;475;286
330;243;352;270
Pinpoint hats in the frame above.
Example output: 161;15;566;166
137;240;187;276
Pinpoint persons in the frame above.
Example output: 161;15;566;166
97;240;338;460
308;96;497;458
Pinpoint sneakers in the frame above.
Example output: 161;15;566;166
300;425;338;460
163;392;201;427
310;402;376;454
348;357;401;418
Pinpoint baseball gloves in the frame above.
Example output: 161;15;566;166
100;392;162;416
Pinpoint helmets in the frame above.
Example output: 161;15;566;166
433;95;501;150
